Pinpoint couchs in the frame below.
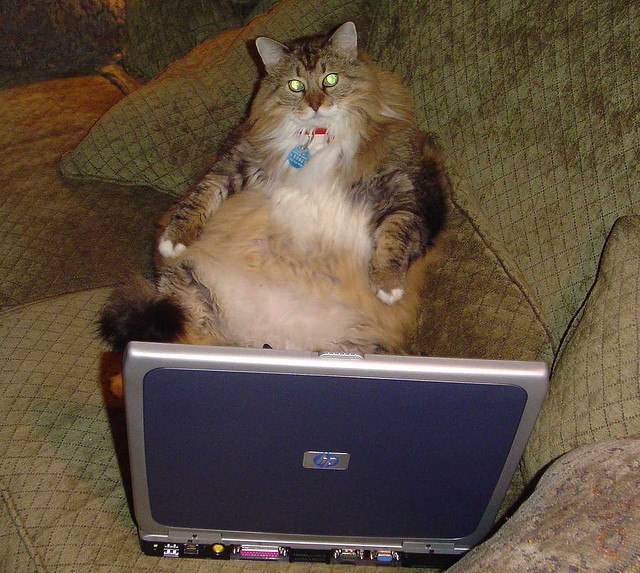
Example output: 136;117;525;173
0;0;640;573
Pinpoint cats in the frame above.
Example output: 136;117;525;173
93;21;449;355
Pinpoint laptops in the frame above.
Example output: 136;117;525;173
121;340;548;568
